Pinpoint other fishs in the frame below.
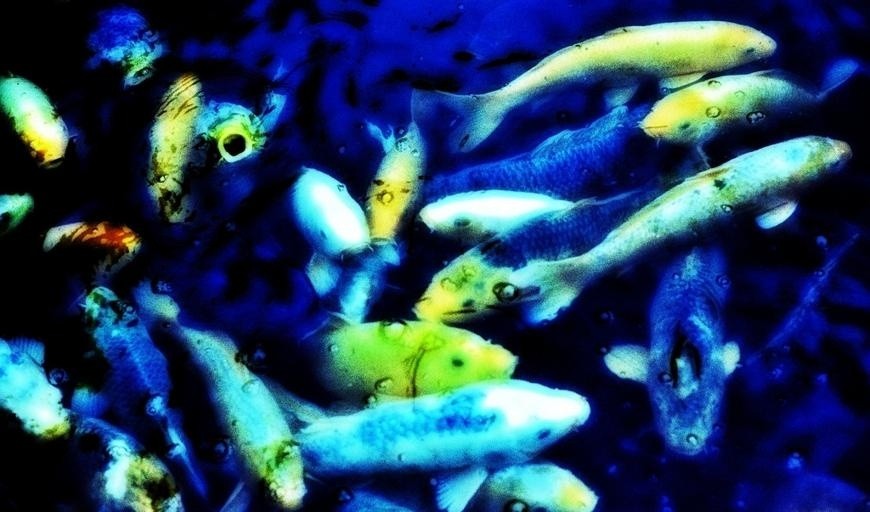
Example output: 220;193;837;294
0;0;870;512
